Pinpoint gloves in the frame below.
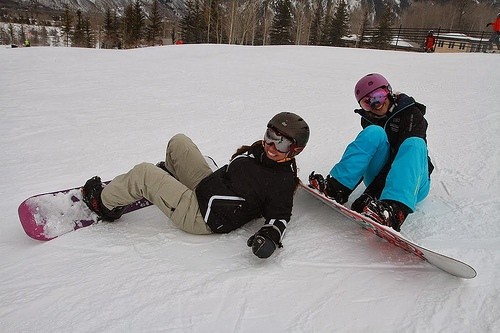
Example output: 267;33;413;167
247;218;288;258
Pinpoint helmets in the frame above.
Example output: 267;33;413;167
354;73;393;107
261;111;310;162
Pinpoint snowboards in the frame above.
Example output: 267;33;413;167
17;156;221;242
297;177;478;279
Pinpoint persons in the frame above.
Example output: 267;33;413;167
309;73;434;233
24;39;31;47
423;32;436;53
485;13;500;53
83;112;310;259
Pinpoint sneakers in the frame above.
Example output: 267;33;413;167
308;171;351;205
363;197;407;231
156;161;179;182
82;175;120;223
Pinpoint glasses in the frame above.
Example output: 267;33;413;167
264;128;292;153
359;88;390;111
